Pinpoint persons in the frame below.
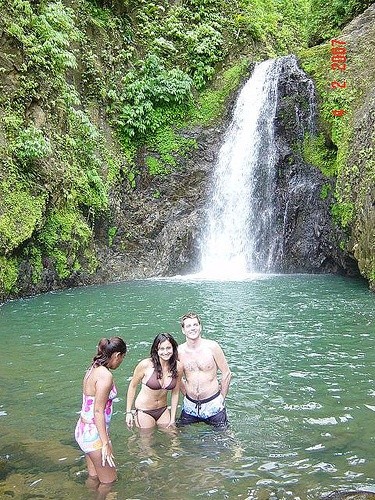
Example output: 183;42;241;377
126;334;179;433
75;337;128;498
176;313;233;431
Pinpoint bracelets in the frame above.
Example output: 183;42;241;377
126;412;131;414
